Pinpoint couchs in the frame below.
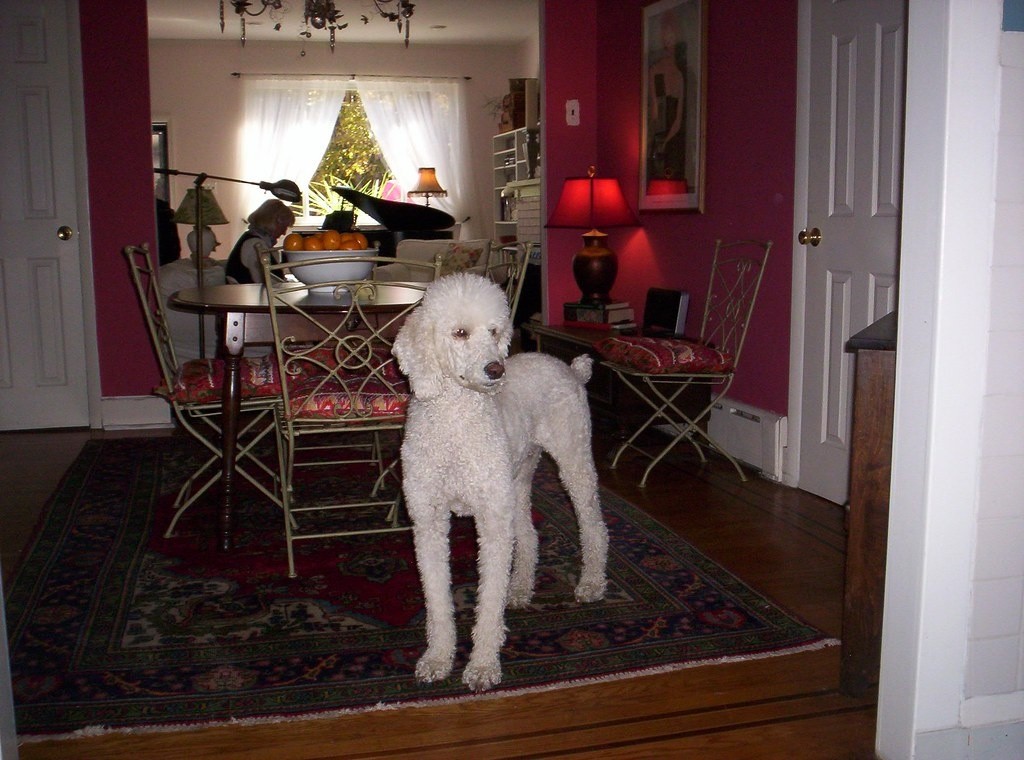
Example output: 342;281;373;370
158;258;272;365
372;239;509;285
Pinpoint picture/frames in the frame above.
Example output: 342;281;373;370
636;0;710;217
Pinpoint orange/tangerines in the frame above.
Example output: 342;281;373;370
283;231;368;251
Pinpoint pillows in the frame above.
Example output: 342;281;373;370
593;335;737;374
283;373;411;425
440;242;484;277
153;358;308;404
288;346;401;378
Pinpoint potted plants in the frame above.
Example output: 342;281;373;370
291;169;397;231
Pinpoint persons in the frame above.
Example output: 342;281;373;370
224;199;295;285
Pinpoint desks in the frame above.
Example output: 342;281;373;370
166;280;428;554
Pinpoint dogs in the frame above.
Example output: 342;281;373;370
390;271;610;697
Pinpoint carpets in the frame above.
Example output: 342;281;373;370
0;429;842;747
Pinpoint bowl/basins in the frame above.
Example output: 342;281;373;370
281;248;378;293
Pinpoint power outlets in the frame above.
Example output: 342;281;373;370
565;99;580;126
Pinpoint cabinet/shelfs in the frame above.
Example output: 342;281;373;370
534;323;676;444
492;123;543;321
292;223;462;267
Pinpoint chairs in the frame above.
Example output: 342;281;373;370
370;239;534;522
124;241;294;540
600;240;772;489
260;253;445;584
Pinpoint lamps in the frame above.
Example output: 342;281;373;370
172;188;230;270
407;167;448;207
153;166;302;203
219;0;416;57
543;165;645;325
